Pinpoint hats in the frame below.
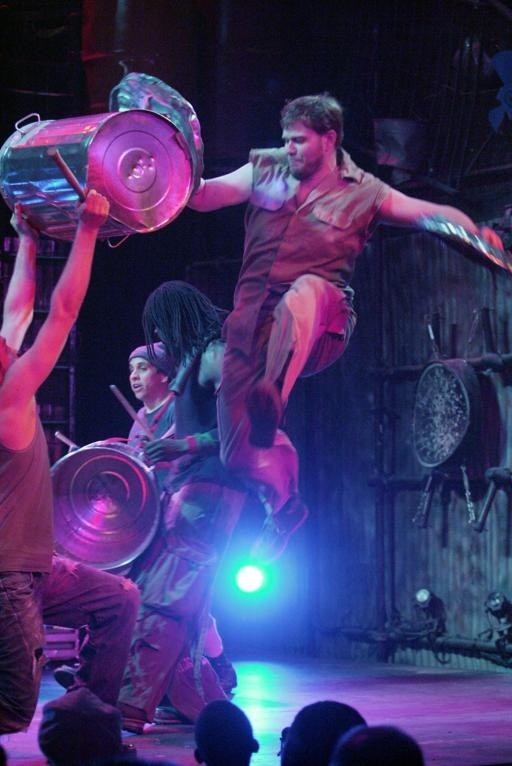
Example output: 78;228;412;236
38;688;122;760
129;341;173;375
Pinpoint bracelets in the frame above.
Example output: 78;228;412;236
187;434;198;455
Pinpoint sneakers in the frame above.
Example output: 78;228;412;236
256;500;309;561
246;380;281;448
55;654;237;736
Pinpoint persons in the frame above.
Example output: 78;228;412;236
34;685;174;766
276;700;366;765
329;722;425;765
123;341;177;466
2;191;149;743
185;93;510;560
193;698;259;766
117;276;239;745
